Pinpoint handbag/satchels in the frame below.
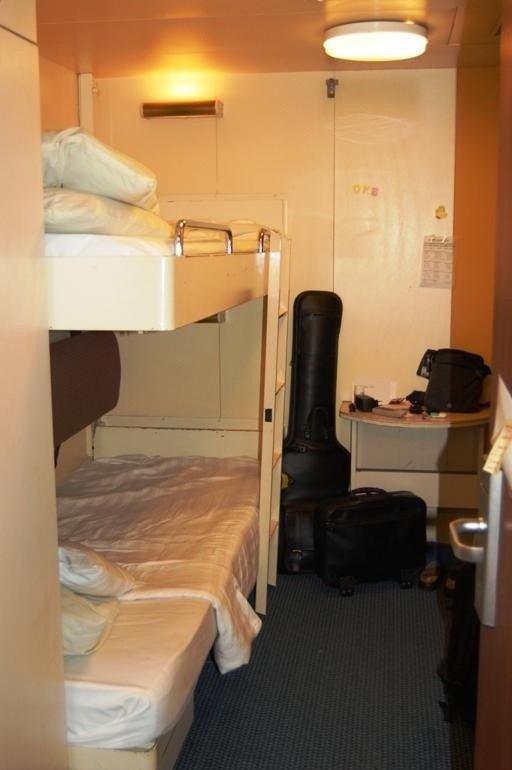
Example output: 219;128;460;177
320;487;427;597
416;348;492;411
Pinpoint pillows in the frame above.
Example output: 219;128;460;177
57;541;135;596
61;588;119;656
42;125;159;208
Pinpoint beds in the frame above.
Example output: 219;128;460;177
43;220;292;770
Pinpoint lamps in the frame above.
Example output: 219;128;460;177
323;21;428;62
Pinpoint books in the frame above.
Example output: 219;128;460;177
372;404;410;419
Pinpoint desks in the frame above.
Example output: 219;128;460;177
338;400;491;515
